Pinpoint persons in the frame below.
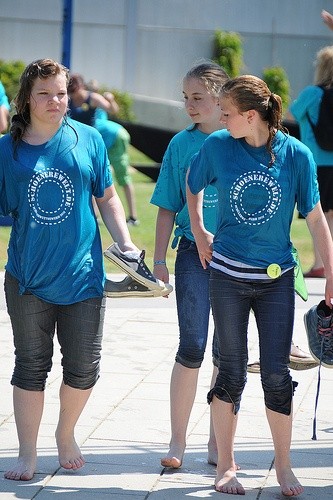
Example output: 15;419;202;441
85;78;108;121
0;80;11;133
0;59;141;480
67;75;119;126
92;118;139;225
149;63;242;471
185;74;333;496
287;45;333;278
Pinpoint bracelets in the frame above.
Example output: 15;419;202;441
153;260;165;265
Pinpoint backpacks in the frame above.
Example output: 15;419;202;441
305;83;333;153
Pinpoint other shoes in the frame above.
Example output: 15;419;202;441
103;275;172;298
288;340;320;370
246;362;261;373
303;269;326;278
304;300;333;368
103;243;165;293
